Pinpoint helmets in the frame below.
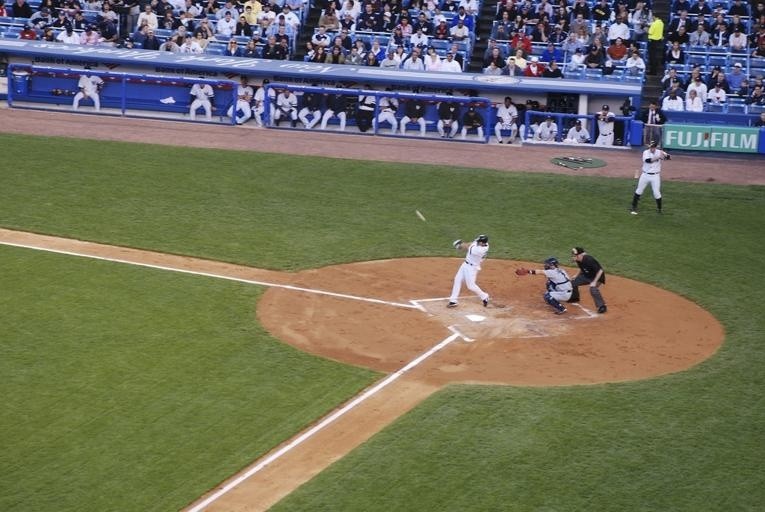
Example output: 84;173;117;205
544;258;558;269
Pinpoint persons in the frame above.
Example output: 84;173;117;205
645;12;664;76
187;76;216;122
446;233;490;308
514;258;574;316
0;0;315;60
73;66;104;110
483;0;655;80
229;75;485;140
305;0;481;73
495;96;635;145
644;103;663;143
661;0;765;127
629;140;672;216
566;247;607;314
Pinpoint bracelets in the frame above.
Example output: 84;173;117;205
666;154;671;159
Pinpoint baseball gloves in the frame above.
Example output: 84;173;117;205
516;268;529;275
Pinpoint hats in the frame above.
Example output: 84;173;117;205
545;105;613;125
262;2;290;21
396;27;435;50
648;140;657;147
670;77;724;89
239;75;479;114
570;245;586;256
475;234;488;241
40;6;92;31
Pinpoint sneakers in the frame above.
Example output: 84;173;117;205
481;293;489;306
556;305;568;315
447;301;457;308
567;296;580;303
599;306;607;313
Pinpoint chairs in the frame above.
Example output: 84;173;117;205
0;0;765;137
